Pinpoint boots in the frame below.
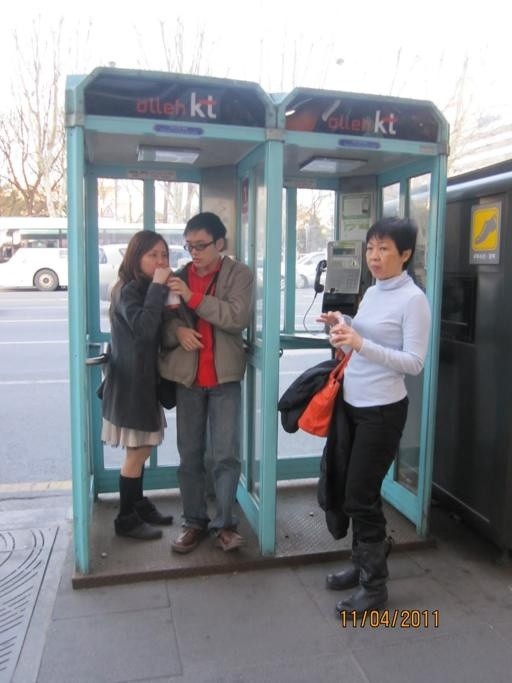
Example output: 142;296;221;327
326;539;390;620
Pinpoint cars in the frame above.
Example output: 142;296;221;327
98;239;328;300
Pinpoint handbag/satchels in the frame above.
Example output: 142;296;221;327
298;346;352;438
158;377;177;409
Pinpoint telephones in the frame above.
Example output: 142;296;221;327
314;260;327;293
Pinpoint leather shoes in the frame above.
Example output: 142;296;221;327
114;496;173;539
171;527;246;553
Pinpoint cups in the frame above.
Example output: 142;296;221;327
163;276;180;308
330;313;352;347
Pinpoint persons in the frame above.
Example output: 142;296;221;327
316;217;431;619
101;230;172;542
158;212;253;554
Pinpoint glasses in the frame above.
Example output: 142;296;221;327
183;240;215;252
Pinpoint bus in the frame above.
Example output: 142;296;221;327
1;216;193;291
